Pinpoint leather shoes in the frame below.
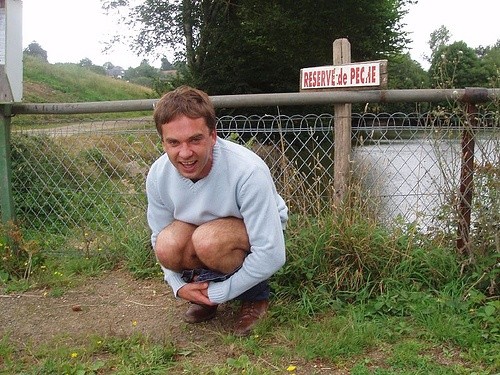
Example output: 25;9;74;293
183;301;219;322
233;301;268;336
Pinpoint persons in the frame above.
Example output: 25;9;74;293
146;85;288;340
